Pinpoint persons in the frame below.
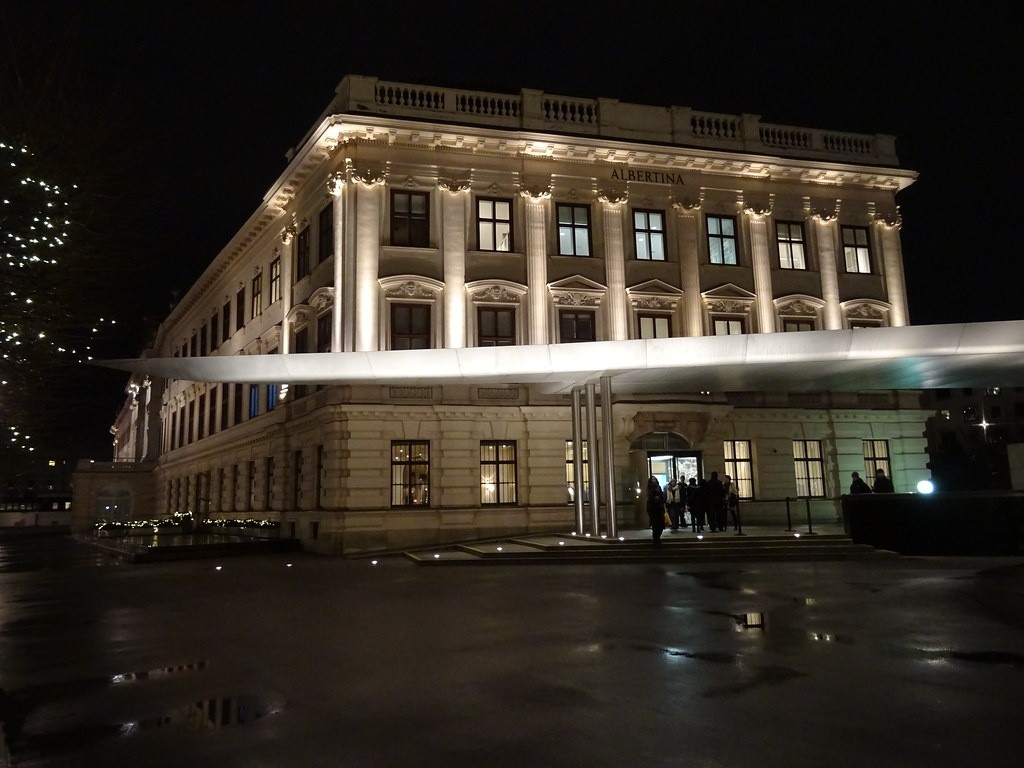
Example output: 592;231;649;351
872;468;894;493
677;475;687;527
848;471;873;494
695;474;709;526
687;478;702;533
663;480;682;533
706;472;723;532
648;493;665;544
722;475;738;531
647;476;662;497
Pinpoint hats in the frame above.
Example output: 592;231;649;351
668;479;677;484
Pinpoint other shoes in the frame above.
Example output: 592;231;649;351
708;530;718;533
720;529;726;531
734;528;737;530
701;528;704;530
697;530;700;533
681;525;688;527
654;541;662;544
693;530;695;532
670;529;679;533
